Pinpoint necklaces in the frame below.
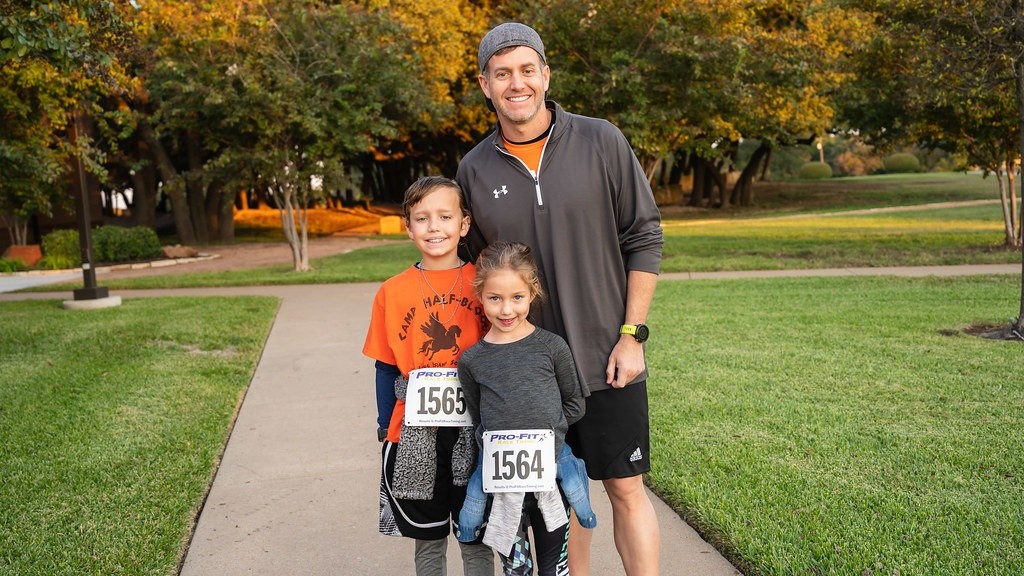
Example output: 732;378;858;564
420;261;461;310
421;257;463;323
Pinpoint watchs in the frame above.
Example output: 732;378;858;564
619;324;650;343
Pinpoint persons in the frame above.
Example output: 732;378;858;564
451;20;666;576
455;241;596;576
361;177;497;576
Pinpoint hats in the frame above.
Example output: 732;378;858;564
479;22;547;75
